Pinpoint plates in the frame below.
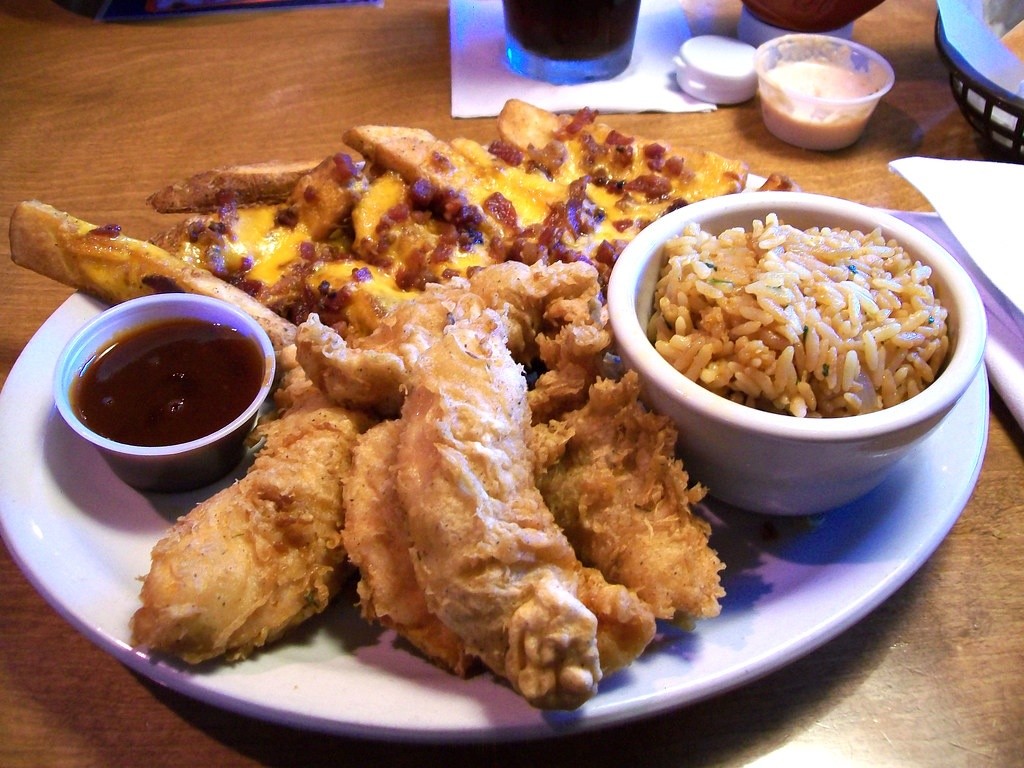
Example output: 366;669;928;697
0;291;988;738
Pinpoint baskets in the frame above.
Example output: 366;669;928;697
934;8;1024;158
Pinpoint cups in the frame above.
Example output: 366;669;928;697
501;1;641;84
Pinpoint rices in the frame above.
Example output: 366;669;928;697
641;210;950;418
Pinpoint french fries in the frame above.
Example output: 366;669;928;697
9;96;801;347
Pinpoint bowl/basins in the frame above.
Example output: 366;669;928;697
53;293;276;492
738;4;853;47
609;191;988;517
755;33;896;151
